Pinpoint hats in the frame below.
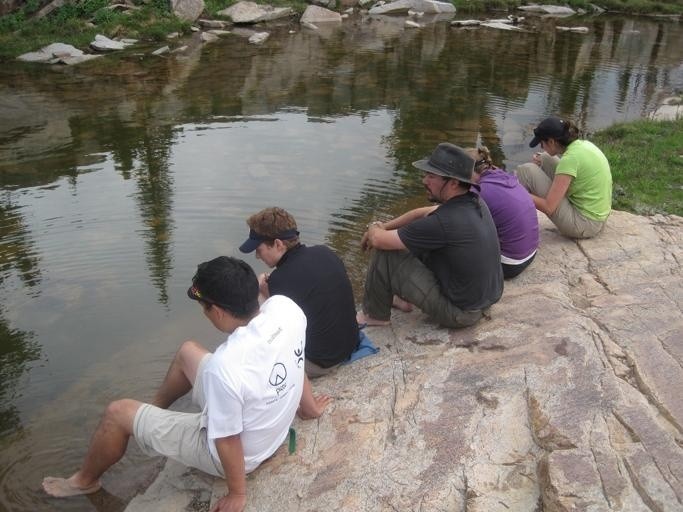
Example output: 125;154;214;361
411;142;482;192
187;280;261;311
529;118;569;147
238;227;300;253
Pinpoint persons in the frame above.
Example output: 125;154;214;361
42;257;330;511
463;146;538;279
517;117;614;240
239;208;359;376
355;142;505;328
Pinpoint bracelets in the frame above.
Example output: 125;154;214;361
365;219;383;228
